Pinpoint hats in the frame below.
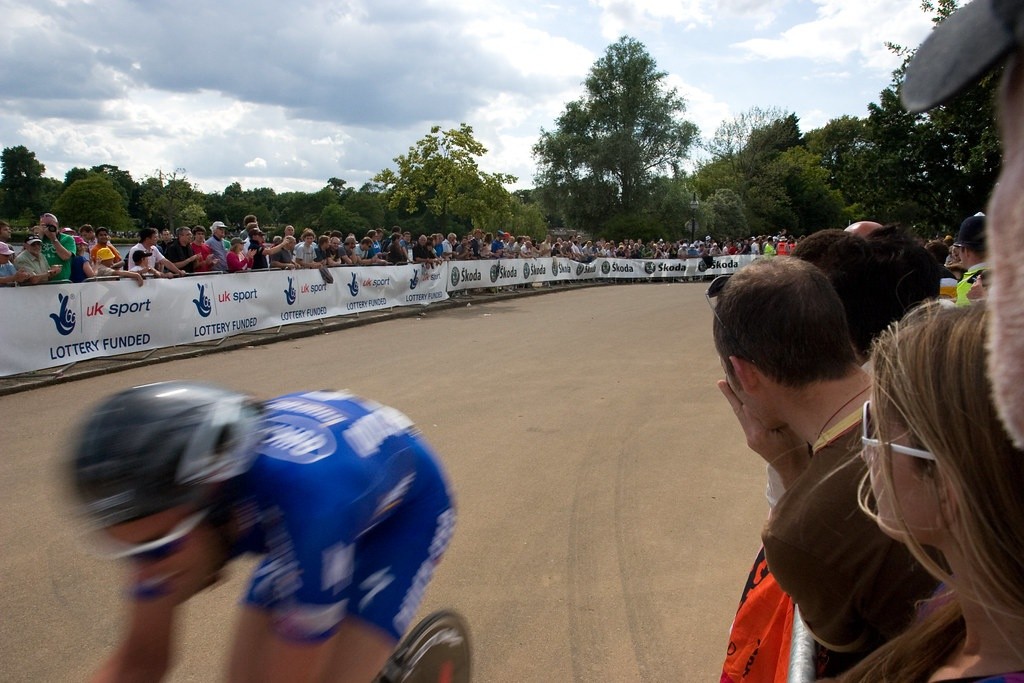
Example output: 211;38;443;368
682;243;687;247
96;246;115;260
706;235;711;240
230;237;246;245
212;221;227;230
248;228;266;237
690;244;694;247
619;245;623;248
344;237;359;244
752;236;756;241
712;243;718;245
0;241;14;255
953;215;986;248
24;233;43;246
131;249;152;262
74;236;90;245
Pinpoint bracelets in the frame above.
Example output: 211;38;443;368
48;271;52;276
50;239;59;244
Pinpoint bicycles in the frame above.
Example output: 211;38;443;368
352;610;475;683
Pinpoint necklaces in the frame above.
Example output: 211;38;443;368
817;383;873;440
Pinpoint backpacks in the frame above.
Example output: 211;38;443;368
381;238;392;253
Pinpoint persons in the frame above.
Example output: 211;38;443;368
898;0;1024;454
0;207;994;308
712;212;1024;683
49;377;461;682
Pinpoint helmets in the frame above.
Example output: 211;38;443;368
73;379;265;529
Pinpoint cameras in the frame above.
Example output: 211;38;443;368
46;224;57;232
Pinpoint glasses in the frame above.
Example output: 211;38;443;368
112;506;213;565
861;396;935;461
702;273;740;359
